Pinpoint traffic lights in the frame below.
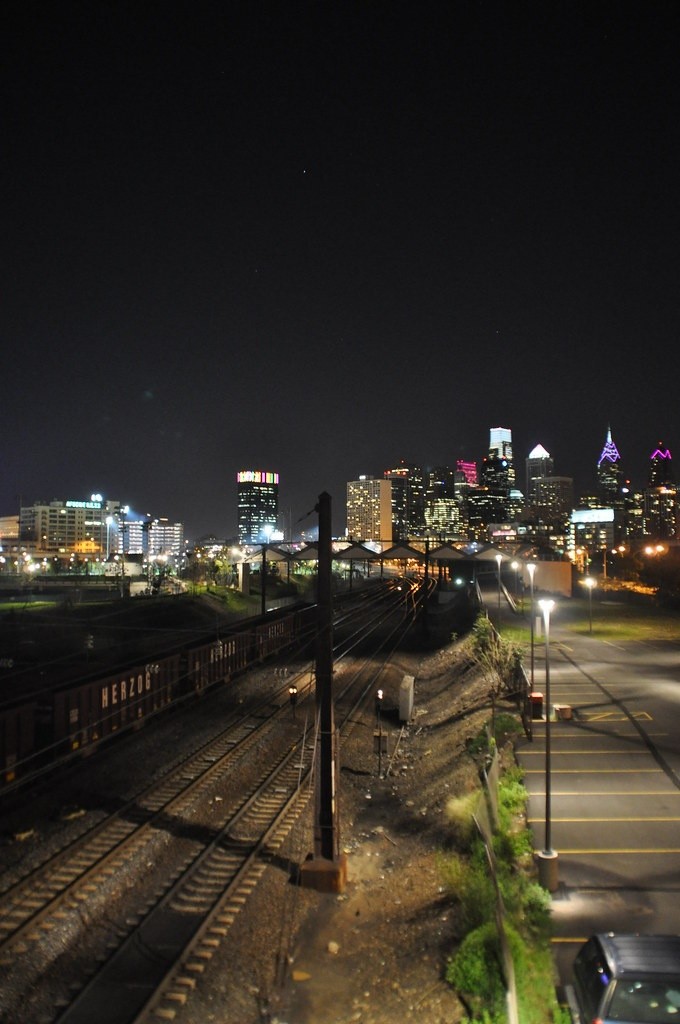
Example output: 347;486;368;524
288;685;298;707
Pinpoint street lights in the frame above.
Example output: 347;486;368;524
576;548;589;577
510;561;519;605
536;599;557;856
495;554;502;633
376;689;384;779
619;546;626;582
1;551;169;579
526;564;535;694
585;578;594;635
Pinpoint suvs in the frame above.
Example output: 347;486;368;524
564;930;680;1024
